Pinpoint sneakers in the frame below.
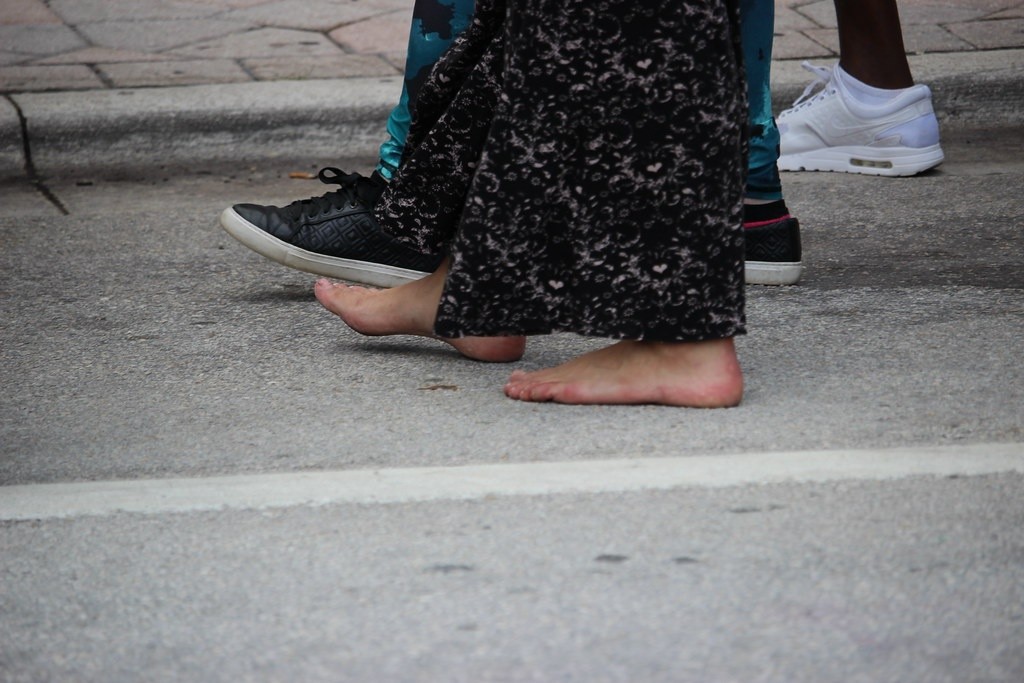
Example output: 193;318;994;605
743;217;803;284
773;59;944;177
219;167;450;291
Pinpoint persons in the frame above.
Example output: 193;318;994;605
222;0;945;411
